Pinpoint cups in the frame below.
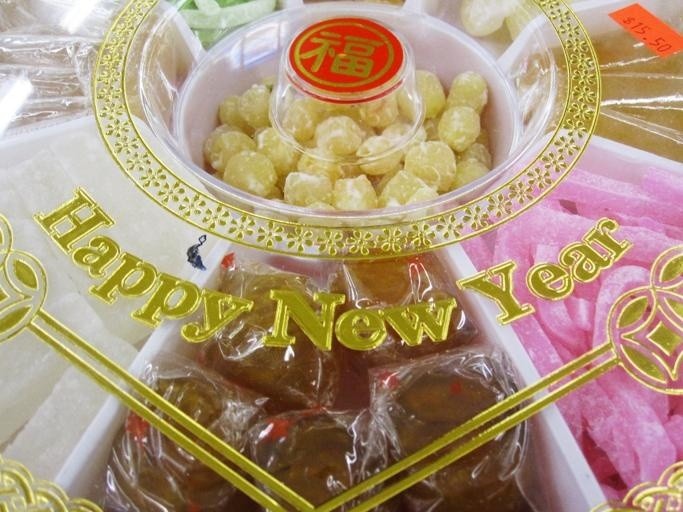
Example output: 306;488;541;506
277;20;423;163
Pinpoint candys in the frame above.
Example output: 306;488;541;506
460;0;506;37
206;72;491;209
